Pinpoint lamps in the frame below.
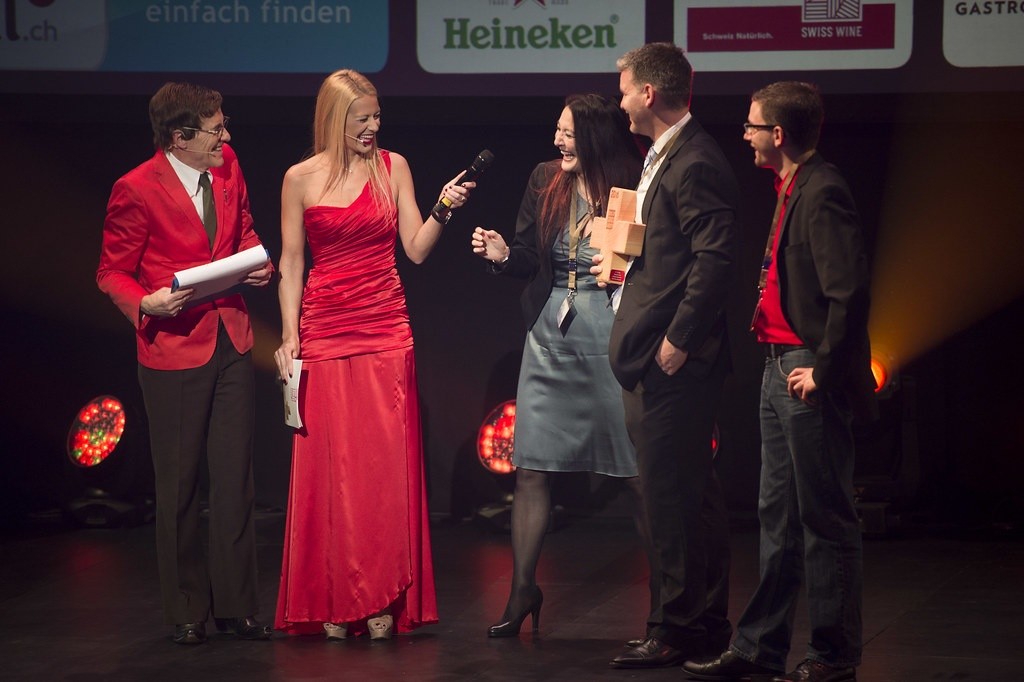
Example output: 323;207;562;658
477;400;574;536
67;393;149;527
851;358;927;541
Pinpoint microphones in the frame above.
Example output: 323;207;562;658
431;150;495;224
184;148;217;157
344;133;363;143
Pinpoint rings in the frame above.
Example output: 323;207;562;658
180;306;182;309
460;196;465;201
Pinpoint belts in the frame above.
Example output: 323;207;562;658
766;343;806;361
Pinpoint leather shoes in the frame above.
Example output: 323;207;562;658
624;638;646;650
773;653;856;682
173;622;207;644
610;636;684;668
215;613;271;640
684;643;782;679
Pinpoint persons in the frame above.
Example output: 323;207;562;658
609;44;740;668
680;81;870;682
96;80;275;643
274;69;477;639
472;91;643;652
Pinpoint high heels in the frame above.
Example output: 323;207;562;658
487;584;543;639
367;605;393;640
324;619;346;640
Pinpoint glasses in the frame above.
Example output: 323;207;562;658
743;118;787;139
178;114;230;142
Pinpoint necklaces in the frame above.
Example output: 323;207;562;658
325;150;359;173
639;119;690;187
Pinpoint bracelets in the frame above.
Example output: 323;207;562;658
433;203;452;225
491;246;508;272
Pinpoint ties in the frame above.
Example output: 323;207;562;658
198;172;218;251
643;147;656;177
